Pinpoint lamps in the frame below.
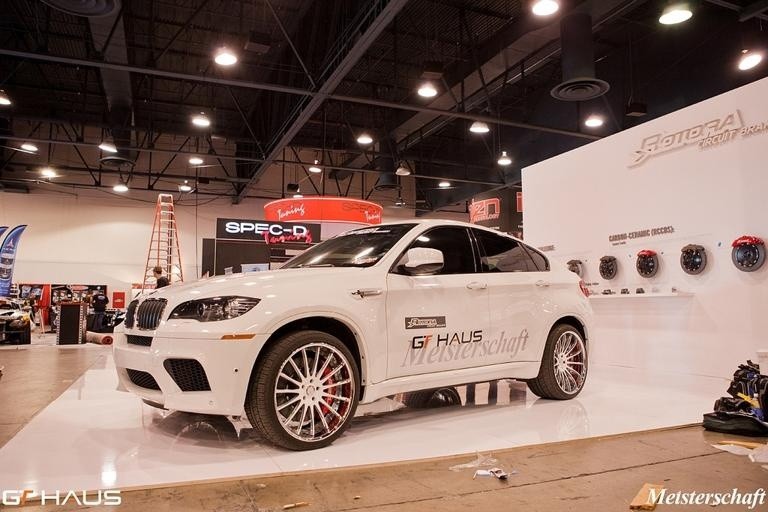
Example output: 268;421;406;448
658;3;693;25
308;79;513;206
95;43;239;200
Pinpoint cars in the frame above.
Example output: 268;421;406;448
107;214;600;454
0;295;32;345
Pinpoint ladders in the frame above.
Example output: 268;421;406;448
142;193;184;294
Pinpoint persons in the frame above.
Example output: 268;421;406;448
87;290;109;332
152;266;169;289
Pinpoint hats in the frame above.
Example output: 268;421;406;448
151;266;163;272
